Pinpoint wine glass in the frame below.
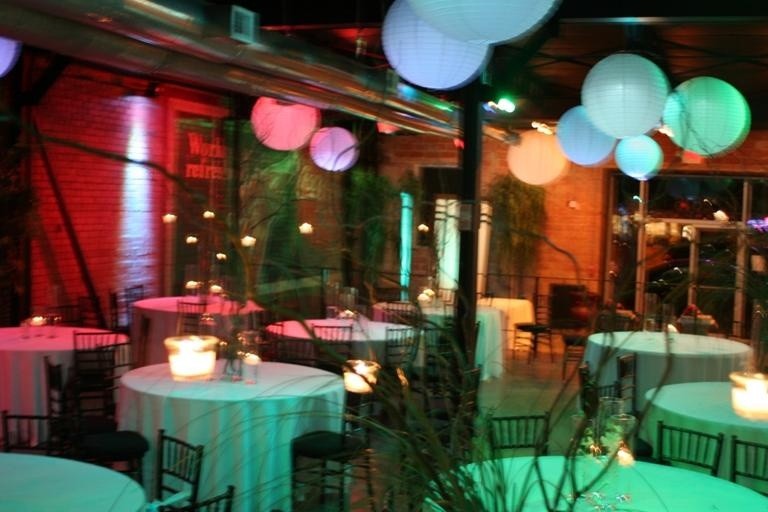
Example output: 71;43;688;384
567;413;597;503
599;395;628;500
607;413;635;503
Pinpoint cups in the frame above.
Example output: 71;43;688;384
243;355;259;386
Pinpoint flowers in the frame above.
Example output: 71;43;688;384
198;311;264;361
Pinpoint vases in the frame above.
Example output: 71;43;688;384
220;358;242;380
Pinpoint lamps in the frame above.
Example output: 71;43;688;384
382;0;488;90
504;128;568;185
0;37;18;75
582;54;668;140
406;0;554;44
614;132;663;178
250;95;320;151
663;76;748;157
309;127;358;172
556;105;617;166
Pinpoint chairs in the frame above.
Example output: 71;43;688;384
384;301;421;330
125;284;145;306
614;351;648;425
380;308;415;327
65;327;117;416
153;429;204;511
512;293;557;365
289;379;378;512
442;304;458;326
257;316;284;363
158;485;235;512
96;341;135;431
174;300;206;338
309;321;352;370
369;323;423;431
45;302;84;327
656;419;724;477
108;291;132;336
43;355;150;486
1;410;66;457
729;433;768;498
749;310;768;370
578;360;644;457
399;363;481;493
562;312;606;380
273;338;319;366
484;410;552;459
423;326;455;377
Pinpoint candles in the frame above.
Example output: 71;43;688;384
727;368;768;421
343;355;380;394
163;335;219;383
